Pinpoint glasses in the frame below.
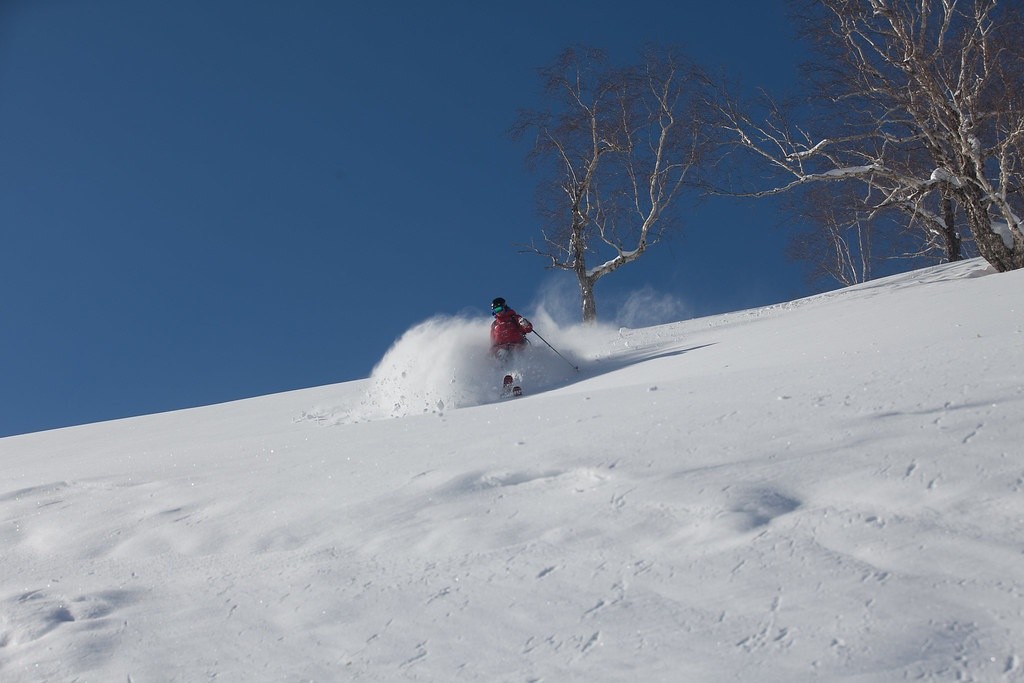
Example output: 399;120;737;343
494;306;503;313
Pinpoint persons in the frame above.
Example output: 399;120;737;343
490;297;533;399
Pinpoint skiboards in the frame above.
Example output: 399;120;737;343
502;375;523;397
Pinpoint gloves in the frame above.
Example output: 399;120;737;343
519;317;530;328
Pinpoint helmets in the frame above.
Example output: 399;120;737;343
491;298;507;310
